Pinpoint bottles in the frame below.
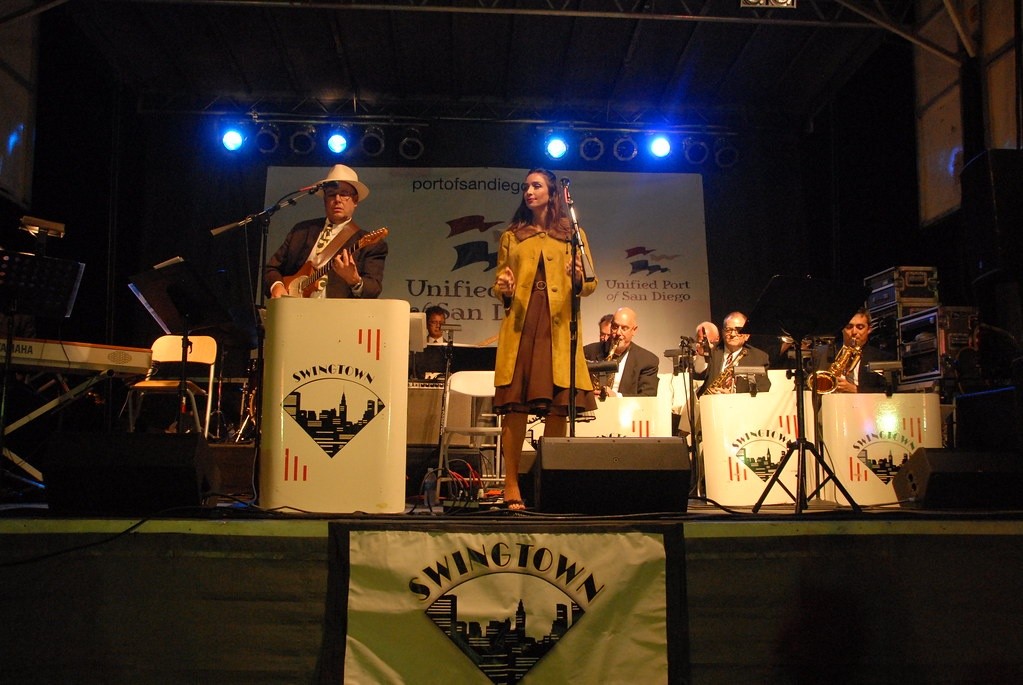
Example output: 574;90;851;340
424;468;437;506
226;423;235;444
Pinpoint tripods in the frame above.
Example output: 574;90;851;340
735;275;863;517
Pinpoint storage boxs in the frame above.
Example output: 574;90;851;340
863;266;981;385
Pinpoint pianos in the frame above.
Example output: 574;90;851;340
2;337;152;488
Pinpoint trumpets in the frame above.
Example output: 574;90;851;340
588;339;620;396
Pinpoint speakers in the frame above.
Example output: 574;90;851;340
953;386;1023;448
523;436;689;520
959;146;1023;284
406;379;475;449
42;430;219;518
892;447;1023;511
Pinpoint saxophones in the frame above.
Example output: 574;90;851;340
698;348;748;398
806;337;863;395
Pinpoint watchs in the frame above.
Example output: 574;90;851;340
352;277;362;290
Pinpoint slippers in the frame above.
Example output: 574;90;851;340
503;499;528;511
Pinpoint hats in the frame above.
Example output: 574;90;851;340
311;164;370;202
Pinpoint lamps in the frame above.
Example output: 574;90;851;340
608;133;638;162
650;130;673;159
679;135;710;165
288;124;319;154
255;121;284;154
577;130;605;162
360;125;386;155
398;127;424;160
327;123;350;153
215;121;252;151
712;136;740;167
542;127;569;158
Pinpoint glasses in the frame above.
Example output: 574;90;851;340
323;189;358;201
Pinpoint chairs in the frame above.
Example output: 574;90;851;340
435;372;526;506
128;334;221;440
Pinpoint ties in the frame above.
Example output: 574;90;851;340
606;355;617;390
846;357;854;386
312;223;332;292
724;353;733;389
433;340;437;343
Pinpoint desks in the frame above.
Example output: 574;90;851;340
523;370;943;507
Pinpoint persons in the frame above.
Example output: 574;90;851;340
945;344;1009;450
837;306;887;393
493;166;598;511
426;306;448;343
678;311;771;496
598;314;614;343
263;163;388;300
584;306;660;398
0;258;37;382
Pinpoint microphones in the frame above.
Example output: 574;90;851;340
321;180;340;189
560;176;570;190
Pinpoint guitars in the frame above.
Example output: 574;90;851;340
282;225;389;298
